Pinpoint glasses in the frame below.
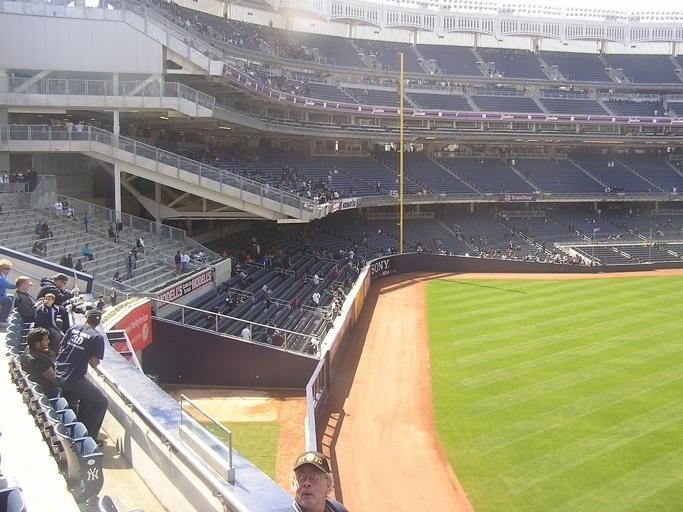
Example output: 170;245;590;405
4;267;10;270
294;471;324;486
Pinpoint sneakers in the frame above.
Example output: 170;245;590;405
0;317;11;327
94;439;103;448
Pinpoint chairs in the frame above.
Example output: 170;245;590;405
1;306;104;512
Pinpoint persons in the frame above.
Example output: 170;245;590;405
266;448;348;512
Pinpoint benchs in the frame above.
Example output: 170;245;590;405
0;193;193;301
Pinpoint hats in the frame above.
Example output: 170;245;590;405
52;273;68;281
293;450;330;476
84;309;102;323
